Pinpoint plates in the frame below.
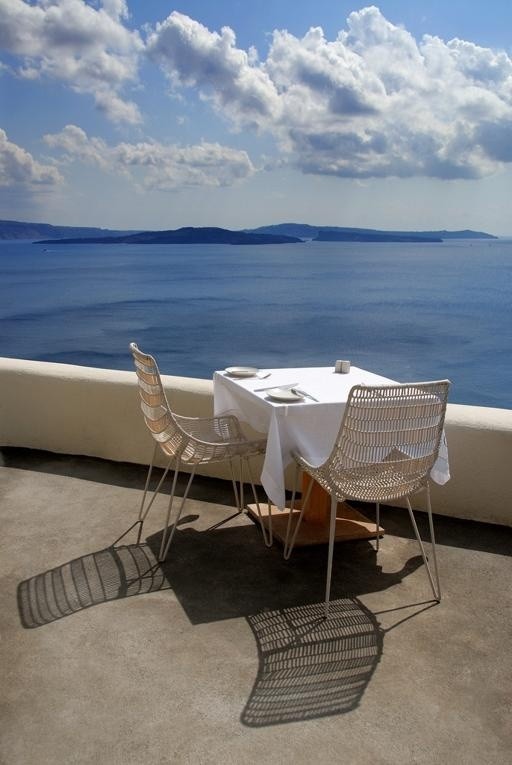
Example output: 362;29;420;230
266;388;305;401
225;366;259;377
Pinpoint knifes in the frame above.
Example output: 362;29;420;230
253;383;299;392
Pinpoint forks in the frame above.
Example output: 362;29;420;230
235;373;271;380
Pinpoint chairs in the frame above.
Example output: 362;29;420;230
284;379;451;618
130;342;273;562
